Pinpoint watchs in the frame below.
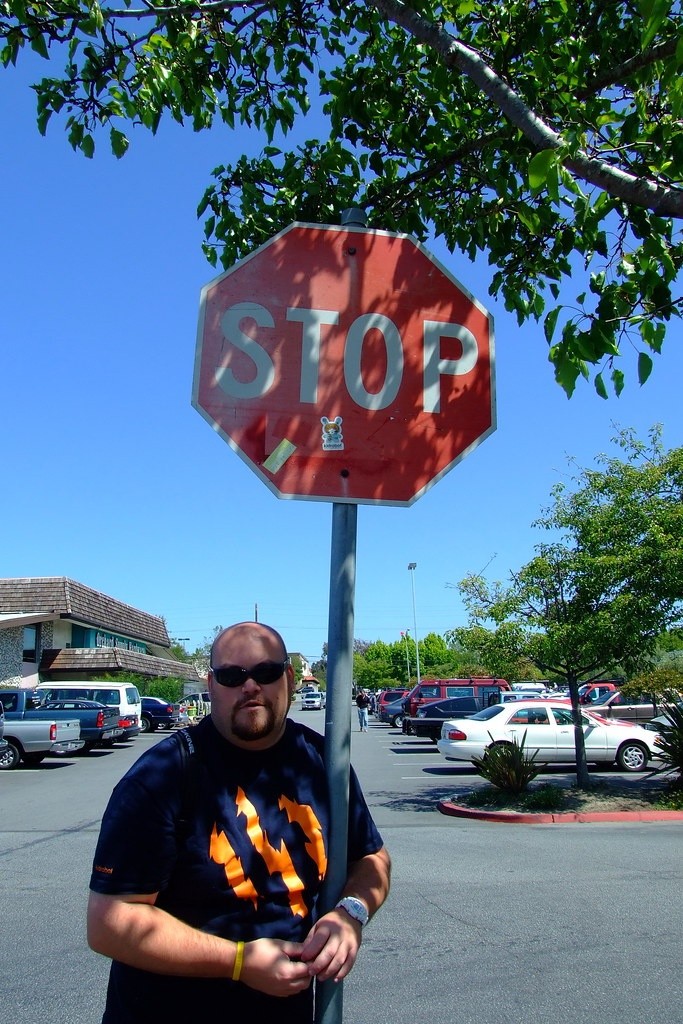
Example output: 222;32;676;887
335;896;369;929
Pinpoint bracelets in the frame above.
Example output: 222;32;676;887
232;941;244;981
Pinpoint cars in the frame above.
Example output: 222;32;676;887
174;692;212;719
436;702;666;772
374;687;437;728
302;692;326;711
580;690;683;727
140;696;188;734
35;700;140;748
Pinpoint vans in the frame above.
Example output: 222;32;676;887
32;680;142;740
401;677;513;718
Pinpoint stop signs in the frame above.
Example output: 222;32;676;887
189;221;497;508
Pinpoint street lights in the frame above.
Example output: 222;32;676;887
405;628;411;682
407;562;420;683
178;638;190;652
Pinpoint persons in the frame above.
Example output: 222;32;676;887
356;689;376;733
87;622;391;1024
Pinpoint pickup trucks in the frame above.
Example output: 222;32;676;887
401;681;622;745
0;690;125;758
0;716;86;770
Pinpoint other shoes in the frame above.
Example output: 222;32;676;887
360;726;363;732
364;729;369;733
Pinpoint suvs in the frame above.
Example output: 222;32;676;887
295;686;314;694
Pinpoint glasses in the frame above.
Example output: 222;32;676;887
208;657;291;689
